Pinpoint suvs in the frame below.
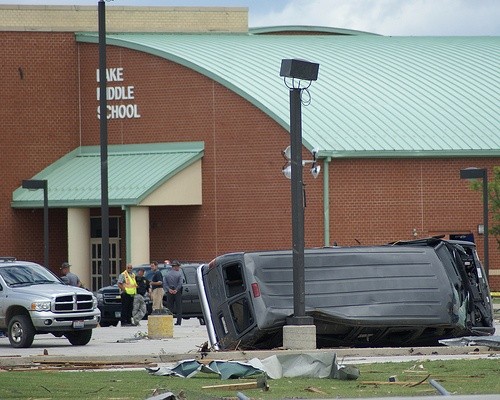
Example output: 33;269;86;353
0;256;102;348
196;236;496;352
94;262;205;326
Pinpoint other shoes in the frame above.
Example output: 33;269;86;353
121;323;137;327
174;323;181;325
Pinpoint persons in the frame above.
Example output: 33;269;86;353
149;261;165;311
163;260;184;326
59;263;81;287
117;263;137;327
132;269;152;326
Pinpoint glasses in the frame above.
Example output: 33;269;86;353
128;267;132;269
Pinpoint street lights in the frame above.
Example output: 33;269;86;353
279;57;320;349
460;167;489;285
21;177;50;270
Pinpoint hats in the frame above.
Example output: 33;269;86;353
60;263;71;269
170;259;180;266
